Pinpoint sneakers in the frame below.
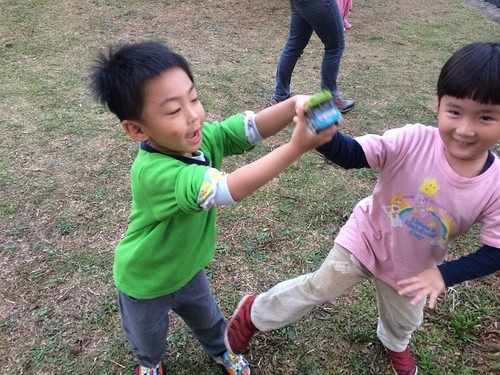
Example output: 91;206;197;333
383;344;418;375
224;294;259;355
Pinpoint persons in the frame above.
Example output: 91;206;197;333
268;0;356;114
224;41;500;375
336;0;353;32
87;40;342;375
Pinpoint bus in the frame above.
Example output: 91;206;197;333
302;90;343;134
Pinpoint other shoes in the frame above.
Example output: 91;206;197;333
269;98;282;107
333;96;355;112
211;349;251;375
134;360;165;375
